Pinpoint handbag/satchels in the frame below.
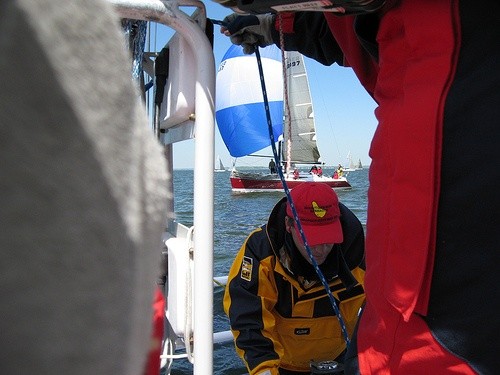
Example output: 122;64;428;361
333;171;338;179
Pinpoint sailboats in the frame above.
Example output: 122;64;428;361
214;157;237;173
214;41;352;195
339;163;364;173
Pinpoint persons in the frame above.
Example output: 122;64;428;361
269;159;276;174
309;165;323;177
294;168;299;179
222;181;367;374
332;164;345;180
219;0;500;375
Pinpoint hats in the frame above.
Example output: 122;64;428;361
287;181;343;247
